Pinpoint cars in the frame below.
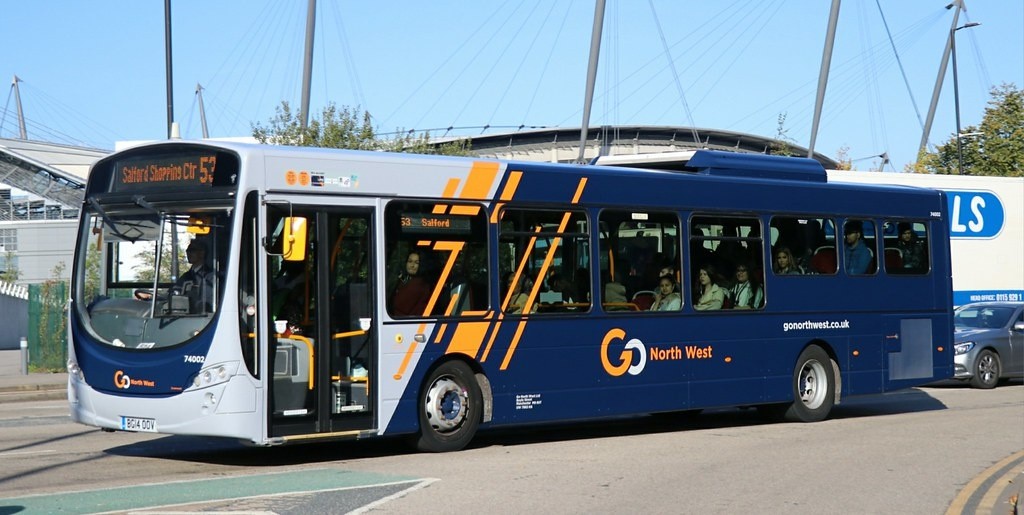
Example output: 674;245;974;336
940;302;1024;390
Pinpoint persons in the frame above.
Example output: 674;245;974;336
135;238;214;313
894;222;922;272
388;250;432;316
502;272;529;314
773;247;802;275
842;220;872;275
274;255;312;316
564;214;837;312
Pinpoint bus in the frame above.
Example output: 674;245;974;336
64;139;957;454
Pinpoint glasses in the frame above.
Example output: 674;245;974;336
185;246;205;254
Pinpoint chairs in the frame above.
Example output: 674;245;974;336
723;287;730;309
630;291;658;311
809;246;904;274
439;276;472;311
